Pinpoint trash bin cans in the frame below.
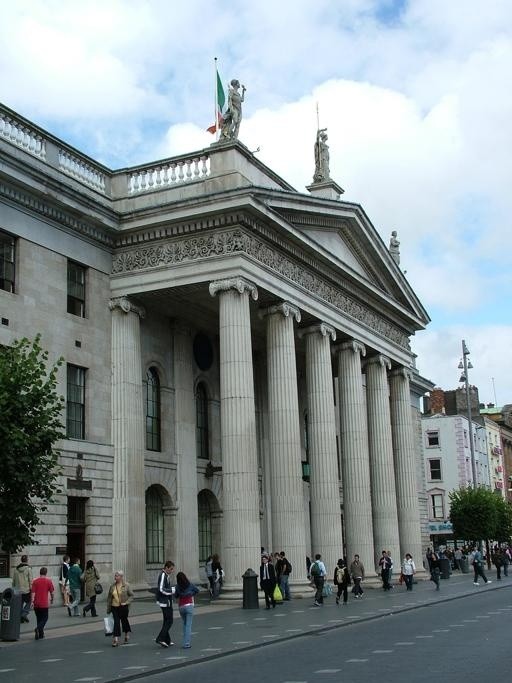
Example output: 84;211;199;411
0;588;22;641
439;558;450;579
460;555;469;573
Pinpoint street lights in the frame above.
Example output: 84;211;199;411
455;338;480;489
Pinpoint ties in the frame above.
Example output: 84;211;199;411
265;565;268;579
383;558;385;569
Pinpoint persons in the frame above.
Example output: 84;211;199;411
12;555;33;624
205;553;224;601
29;567;55;640
155;561;176;648
314;128;330;179
63;558;83;617
174;572;199;648
59;556;72;606
106;570;135;646
80;560;101;618
259;542;512;610
389;231;400;251
227;79;245;138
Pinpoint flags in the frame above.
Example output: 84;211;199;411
207;70;225;134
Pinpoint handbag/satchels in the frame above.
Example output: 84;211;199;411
94;583;103;594
322;580;332;597
104;615;114;636
61;576;70;585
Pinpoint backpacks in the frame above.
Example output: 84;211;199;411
336;566;346;583
311;561;322;576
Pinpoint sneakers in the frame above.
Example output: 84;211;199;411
473;582;480;585
336;600;339;604
111;637;130;646
64;602;99;617
360;593;364;598
354;595;358;599
35;627;44;639
21;614;30;623
313;601;323;606
157;640;191;648
486;581;492;584
